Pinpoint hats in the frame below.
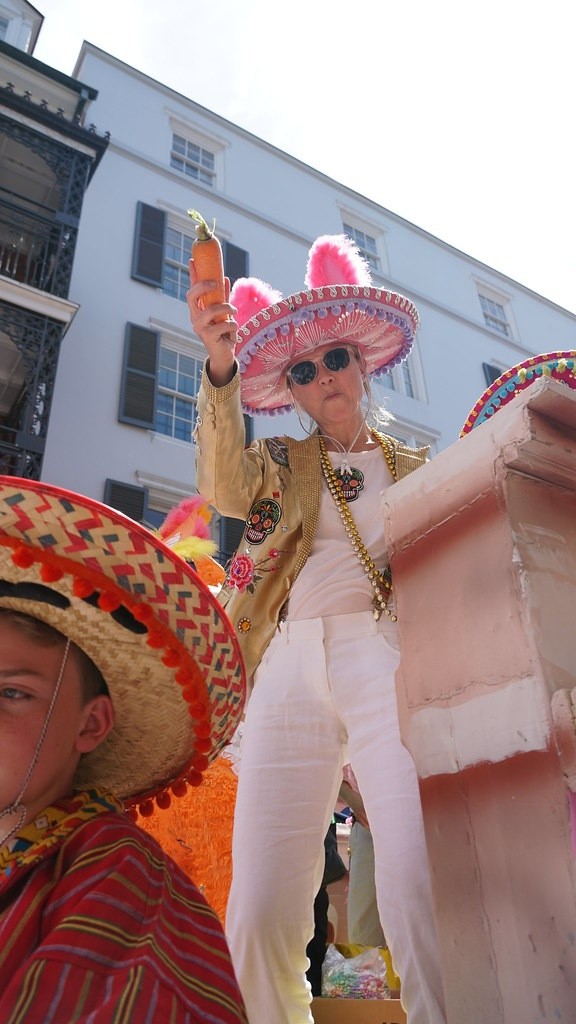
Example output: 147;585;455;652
229;233;421;418
0;475;249;822
457;350;576;437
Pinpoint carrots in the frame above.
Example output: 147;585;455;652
187;209;227;325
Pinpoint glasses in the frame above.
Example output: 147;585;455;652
286;346;356;387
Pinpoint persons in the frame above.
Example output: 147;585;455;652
306;764;386;999
0;475;249;1024
114;497;239;937
187;258;450;1023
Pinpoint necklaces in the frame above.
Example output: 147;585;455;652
319;425;399;619
